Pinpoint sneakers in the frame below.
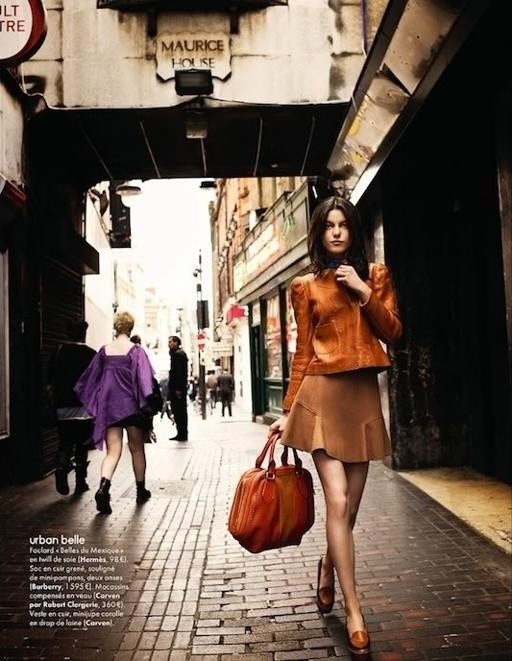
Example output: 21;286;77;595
55;467;70;495
74;478;89;495
169;436;188;441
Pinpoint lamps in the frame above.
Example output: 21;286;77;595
175;67;215;96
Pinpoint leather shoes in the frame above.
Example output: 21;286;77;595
316;558;335;614
345;612;372;659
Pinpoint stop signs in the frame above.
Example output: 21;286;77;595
197;334;205;350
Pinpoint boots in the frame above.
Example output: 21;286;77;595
135;478;151;505
94;476;113;515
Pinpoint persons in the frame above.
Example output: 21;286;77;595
129;334;155;445
159;368;235;425
46;321;100;496
265;195;406;656
72;311;159;516
164;336;189;442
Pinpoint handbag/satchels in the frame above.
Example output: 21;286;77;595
228;430;316;554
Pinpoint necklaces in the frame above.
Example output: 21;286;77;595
325;256;342;268
